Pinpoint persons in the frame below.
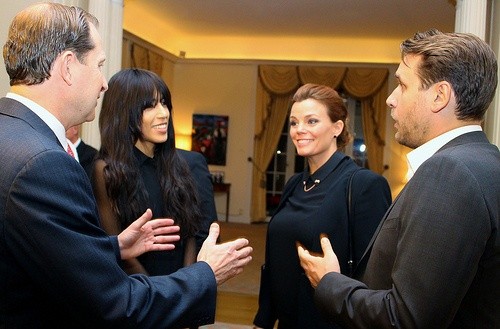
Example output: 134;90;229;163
253;82;392;329
297;29;500;329
65;68;220;280
0;3;252;329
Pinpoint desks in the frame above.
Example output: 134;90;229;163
211;183;231;222
